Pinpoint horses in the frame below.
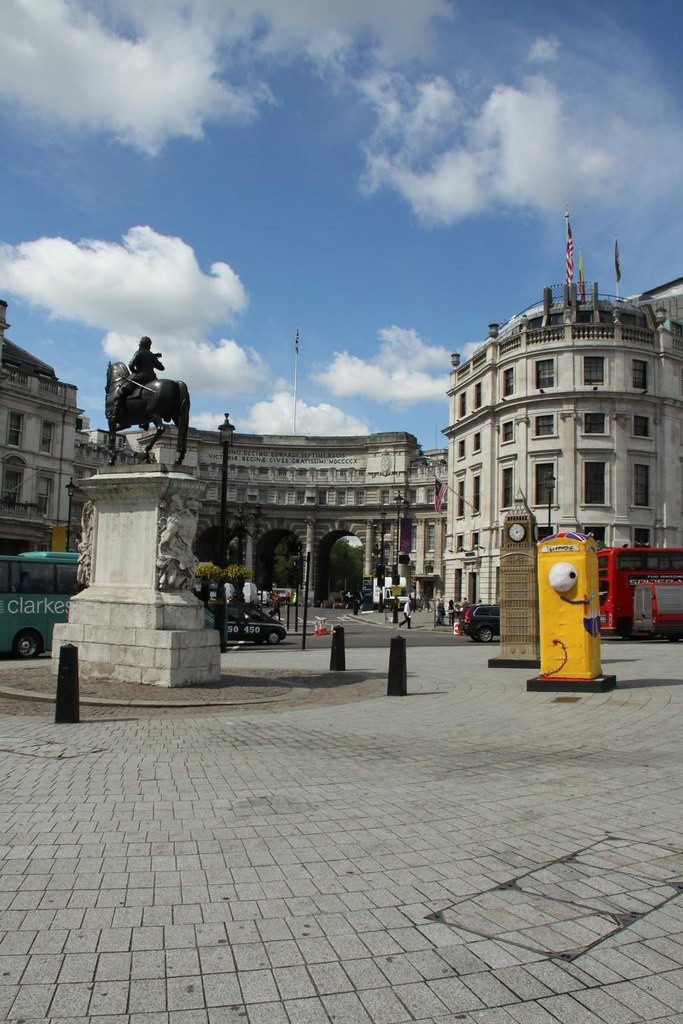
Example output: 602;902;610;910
105;361;191;467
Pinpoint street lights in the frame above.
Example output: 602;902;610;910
544;471;556;536
371;523;387;613
655;502;667;548
217;411;235;653
391;491;405;624
64;475;78;552
293;551;311;651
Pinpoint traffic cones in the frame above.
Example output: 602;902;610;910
313;619;345;637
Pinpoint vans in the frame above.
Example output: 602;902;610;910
210;602;287;645
257;587;298;607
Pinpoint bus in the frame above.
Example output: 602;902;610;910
596;546;683;642
0;551;89;659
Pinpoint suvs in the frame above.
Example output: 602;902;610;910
458;603;500;643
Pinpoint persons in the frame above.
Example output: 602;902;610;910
399;599;412;629
292;592;297;606
462;597;470;605
436;598;444;626
439;598;446;626
478;599;482;603
269;595;283;621
108;336;165;430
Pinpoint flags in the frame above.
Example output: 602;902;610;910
434;479;447;514
579;249;585;302
614;239;621;282
565;224;574;303
